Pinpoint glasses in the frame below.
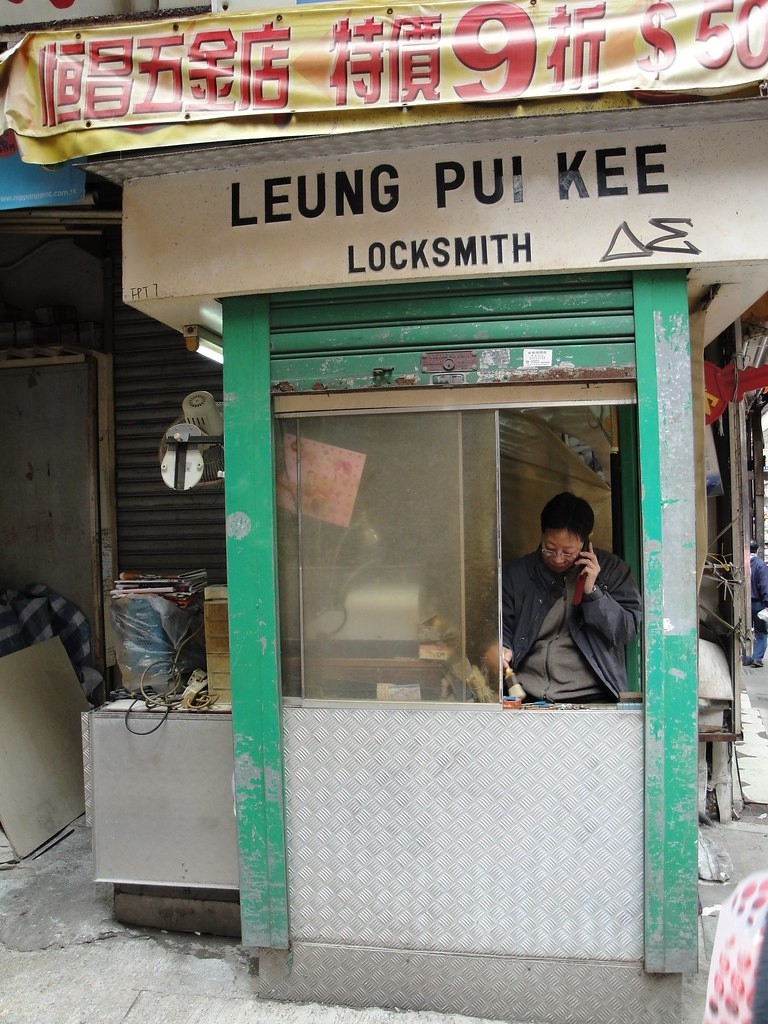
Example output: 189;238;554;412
540;534;583;560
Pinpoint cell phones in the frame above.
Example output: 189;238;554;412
578;536;590;577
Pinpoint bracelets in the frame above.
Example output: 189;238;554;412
590;585;597;594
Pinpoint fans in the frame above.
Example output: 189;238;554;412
159;390;223;488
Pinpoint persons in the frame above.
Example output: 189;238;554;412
475;492;643;704
742;539;768;666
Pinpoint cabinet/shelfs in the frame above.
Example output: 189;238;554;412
204;599;230;706
81;694;301;886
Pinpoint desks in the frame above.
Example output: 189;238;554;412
281;640;467;702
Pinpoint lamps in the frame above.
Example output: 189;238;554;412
742;326;768;366
326;509;388;609
183;325;223;366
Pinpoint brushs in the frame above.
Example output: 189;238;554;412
502;661;526;701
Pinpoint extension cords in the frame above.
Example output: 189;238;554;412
181;678;208;708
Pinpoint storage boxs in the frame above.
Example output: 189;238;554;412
698;698;724;731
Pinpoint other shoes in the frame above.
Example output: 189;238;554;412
752;660;764;667
